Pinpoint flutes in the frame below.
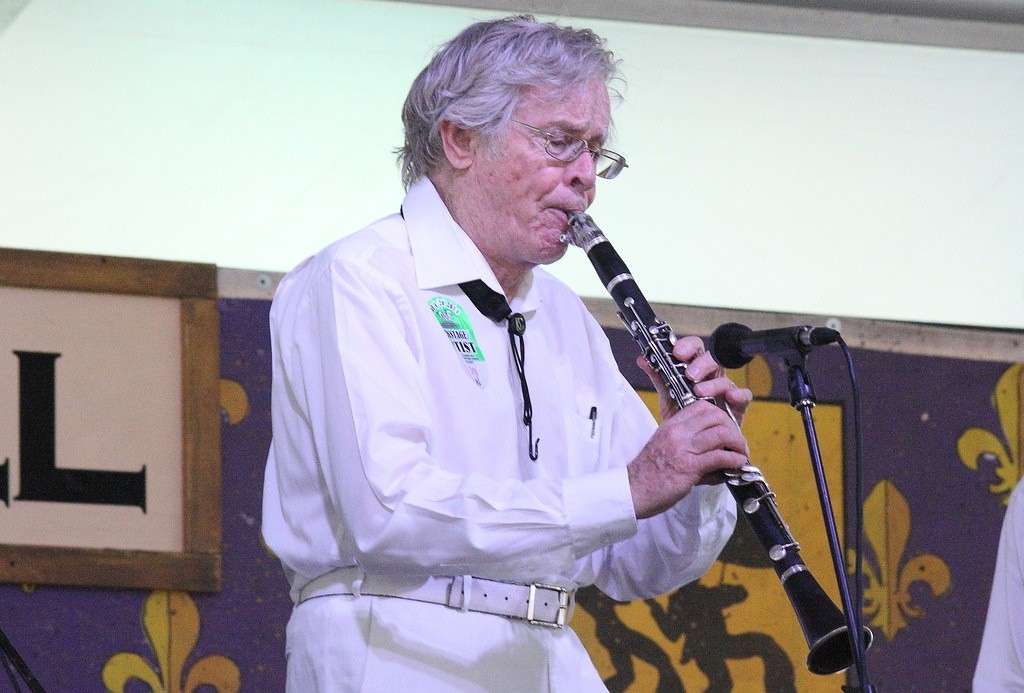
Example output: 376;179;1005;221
556;206;875;678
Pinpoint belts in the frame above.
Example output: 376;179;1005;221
298;566;568;629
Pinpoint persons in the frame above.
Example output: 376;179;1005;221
972;474;1024;693
261;13;751;693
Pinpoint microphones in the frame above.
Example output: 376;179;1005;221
706;322;840;370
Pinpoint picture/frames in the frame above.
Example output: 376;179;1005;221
0;246;224;592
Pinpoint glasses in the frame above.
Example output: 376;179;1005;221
510;118;629;180
